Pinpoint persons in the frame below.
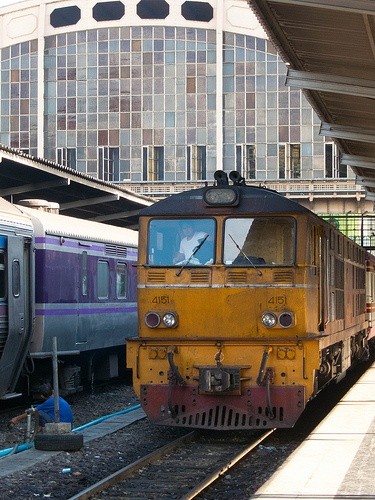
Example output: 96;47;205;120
171;221;213;265
10;383;74;431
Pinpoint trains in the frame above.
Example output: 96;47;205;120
0;195;140;417
124;169;374;434
